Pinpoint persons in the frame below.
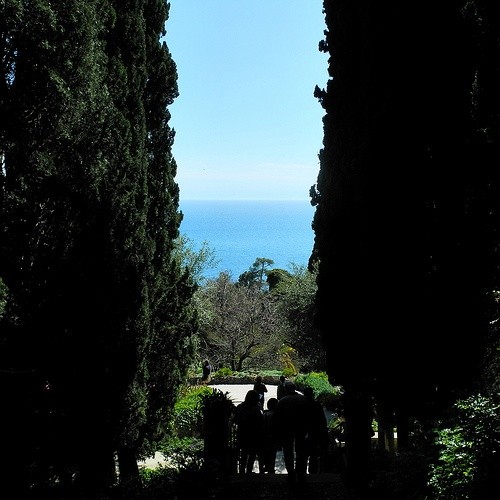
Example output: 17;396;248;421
277;376;286;400
202;360;211;386
234;384;395;479
254;376;268;411
